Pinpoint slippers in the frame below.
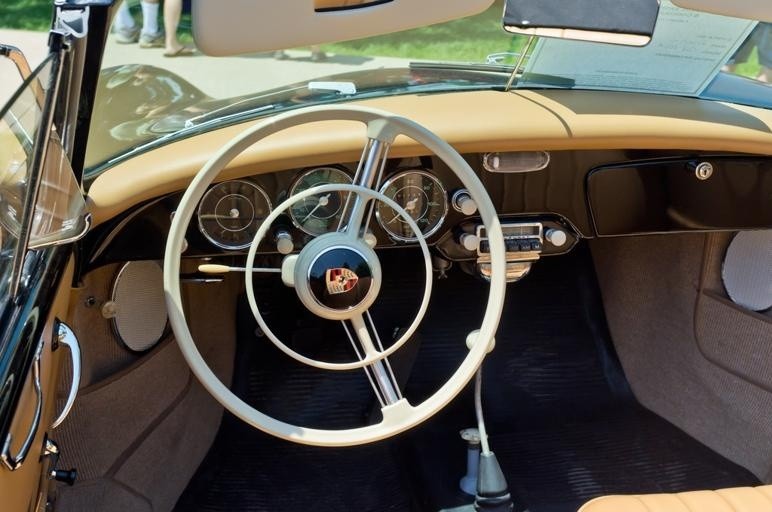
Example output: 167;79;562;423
161;47;196;59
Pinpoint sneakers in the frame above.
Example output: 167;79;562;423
113;30;138;43
138;33;163;48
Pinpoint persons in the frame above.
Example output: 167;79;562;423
163;1;198;58
721;22;772;86
112;1;166;50
